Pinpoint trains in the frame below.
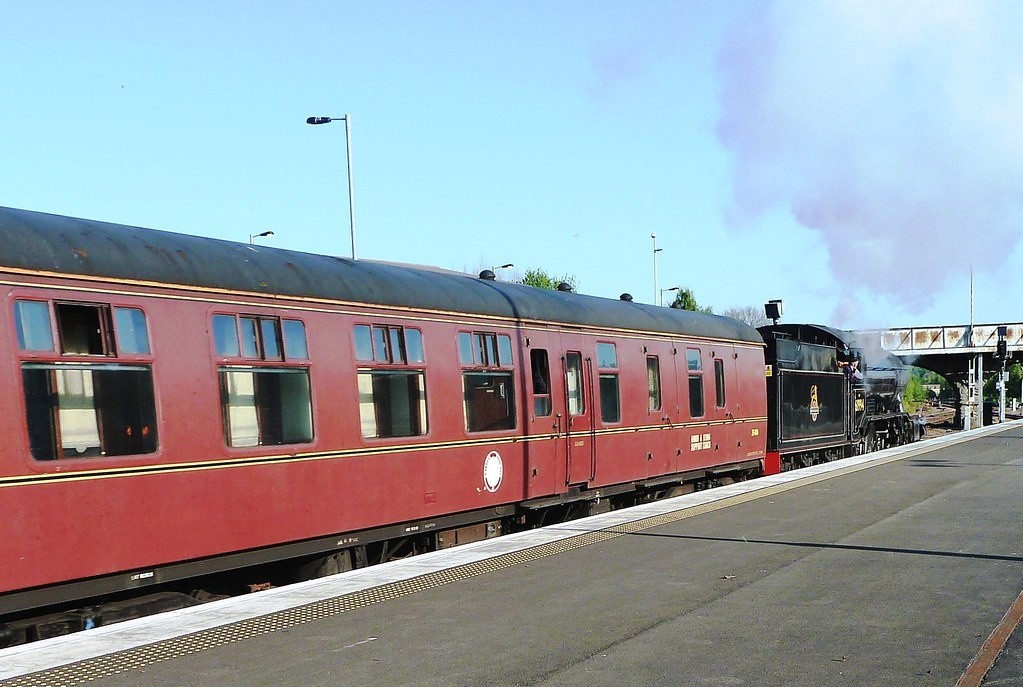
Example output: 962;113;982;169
0;205;929;646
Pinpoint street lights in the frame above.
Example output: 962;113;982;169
765;298;784;324
306;114;358;260
493;264;514;274
248;230;273;246
651;233;663;306
660;287;679;308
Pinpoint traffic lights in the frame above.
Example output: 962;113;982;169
997;340;1008;357
998;326;1007;335
